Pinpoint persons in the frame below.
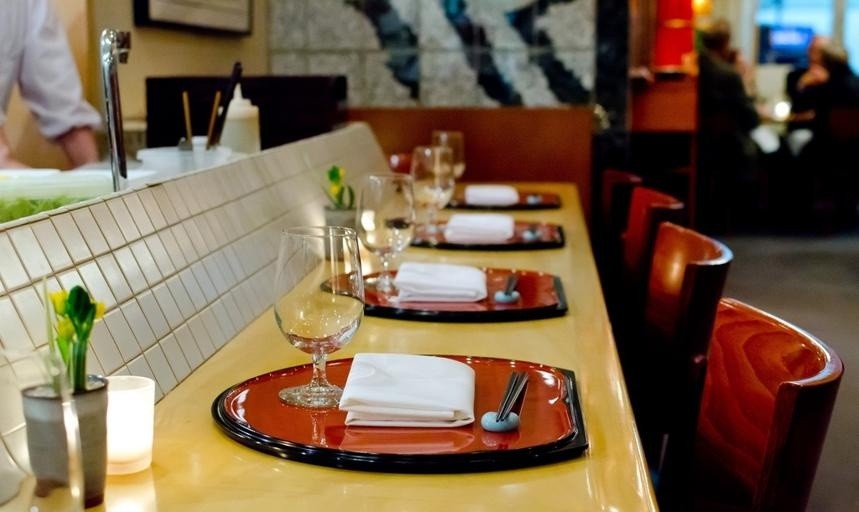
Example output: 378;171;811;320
699;20;763;235
785;35;859;238
0;0;102;169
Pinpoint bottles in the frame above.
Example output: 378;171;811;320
219;83;261;157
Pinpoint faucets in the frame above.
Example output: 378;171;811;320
100;29;131;195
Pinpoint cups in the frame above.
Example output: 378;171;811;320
102;375;156;476
138;143;231;177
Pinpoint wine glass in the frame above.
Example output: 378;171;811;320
354;172;417;296
431;131;466;204
0;350;86;512
411;146;455;236
273;225;365;409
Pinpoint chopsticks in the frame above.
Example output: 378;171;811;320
183;90;221;145
496;369;529;423
504;275;519;296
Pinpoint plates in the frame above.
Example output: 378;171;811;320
385;217;565;252
320;266;568;323
212;352;590;473
445;190;562;210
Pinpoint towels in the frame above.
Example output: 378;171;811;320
337;352;477;431
445;213;514;244
465;185;518;206
393;261;490;304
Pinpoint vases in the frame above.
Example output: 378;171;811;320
318;164;356;260
17;281;108;506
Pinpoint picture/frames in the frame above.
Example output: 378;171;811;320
133;1;254;38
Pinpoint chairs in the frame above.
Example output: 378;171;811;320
629;221;730;402
598;170;639;234
599;187;684;320
661;297;847;512
